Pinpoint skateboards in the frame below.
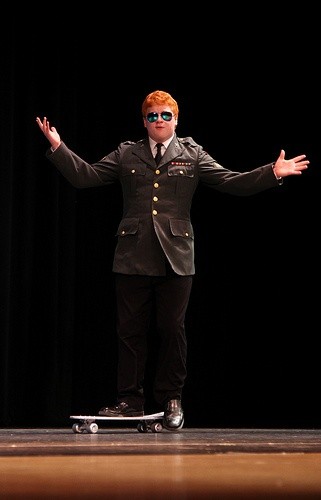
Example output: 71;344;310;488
70;412;165;434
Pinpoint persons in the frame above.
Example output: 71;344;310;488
36;90;309;431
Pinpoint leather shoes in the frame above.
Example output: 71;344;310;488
99;402;144;416
163;399;184;431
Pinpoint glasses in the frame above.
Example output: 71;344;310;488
145;111;175;123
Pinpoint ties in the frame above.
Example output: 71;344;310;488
155;143;164;163
